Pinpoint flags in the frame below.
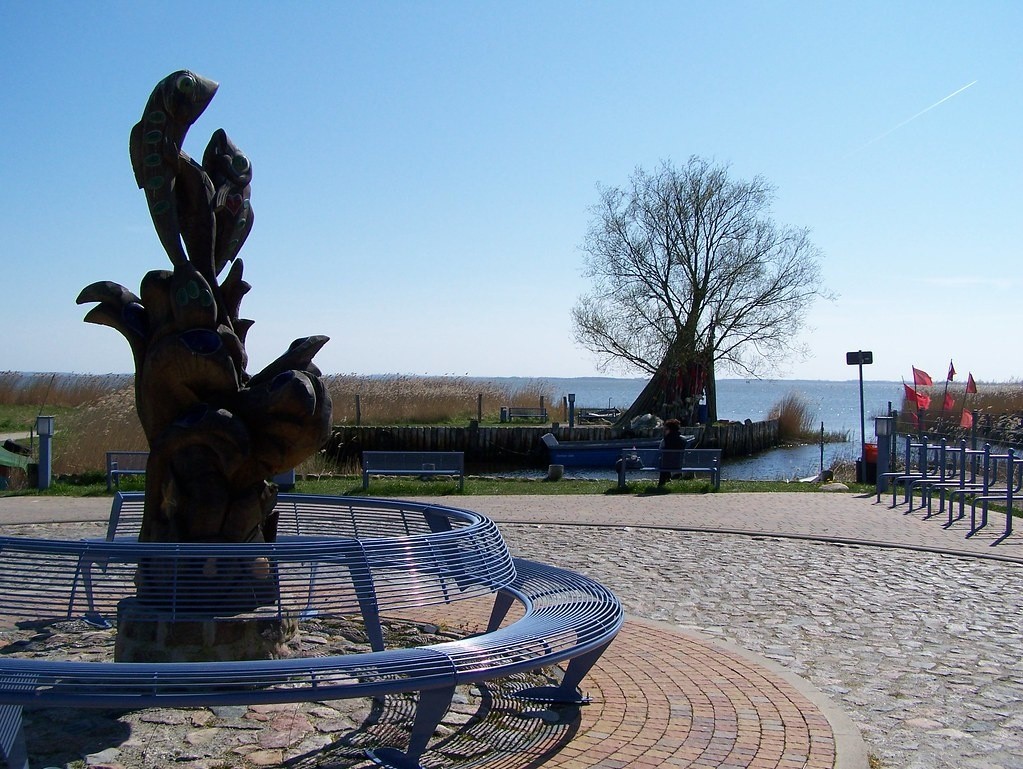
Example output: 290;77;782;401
905;385;920;401
961;409;972;429
944;393;954;409
912;413;926;430
917;393;932;410
948;363;956;381
914;368;932;386
965;374;977;393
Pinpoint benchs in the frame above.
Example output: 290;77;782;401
362;450;467;492
617;448;723;490
106;451;149;490
577;408;622;426
508;406;547;423
0;492;624;769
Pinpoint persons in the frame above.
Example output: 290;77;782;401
657;419;686;487
